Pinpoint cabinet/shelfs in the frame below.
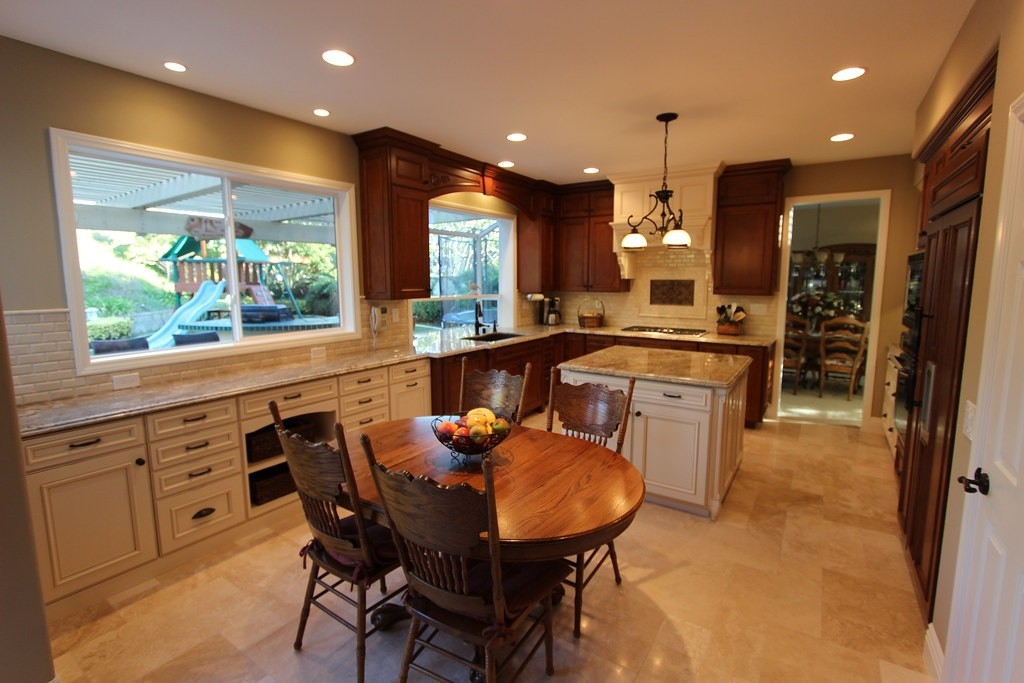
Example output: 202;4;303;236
434;330;774;516
880;352;902;456
356;137;629;300
18;357;429;658
712;158;784;296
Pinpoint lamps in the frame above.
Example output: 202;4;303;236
789;204;847;278
620;112;691;251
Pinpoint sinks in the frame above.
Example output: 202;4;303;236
460;332;525;342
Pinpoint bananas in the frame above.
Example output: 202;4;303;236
467;407;496;424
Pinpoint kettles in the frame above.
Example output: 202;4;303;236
546;309;561;326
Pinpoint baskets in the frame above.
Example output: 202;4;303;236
717;321;740;334
577;297;606;326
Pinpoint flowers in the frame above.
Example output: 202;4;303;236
789;290;860;322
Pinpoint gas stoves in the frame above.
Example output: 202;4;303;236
621;325;708;338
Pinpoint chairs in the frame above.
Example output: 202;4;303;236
265;400;429;683
546;365;636;641
781;313;810;395
815;316;871;403
358;430;575;683
457;356;532;426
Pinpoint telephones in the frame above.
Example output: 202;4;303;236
371;305;389;331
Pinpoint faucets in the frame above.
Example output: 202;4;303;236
475;302;489;335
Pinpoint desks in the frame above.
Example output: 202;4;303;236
793;331;872;385
325;415;647;676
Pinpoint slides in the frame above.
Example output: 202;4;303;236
146;279;226;350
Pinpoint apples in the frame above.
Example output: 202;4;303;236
435;415;511;452
